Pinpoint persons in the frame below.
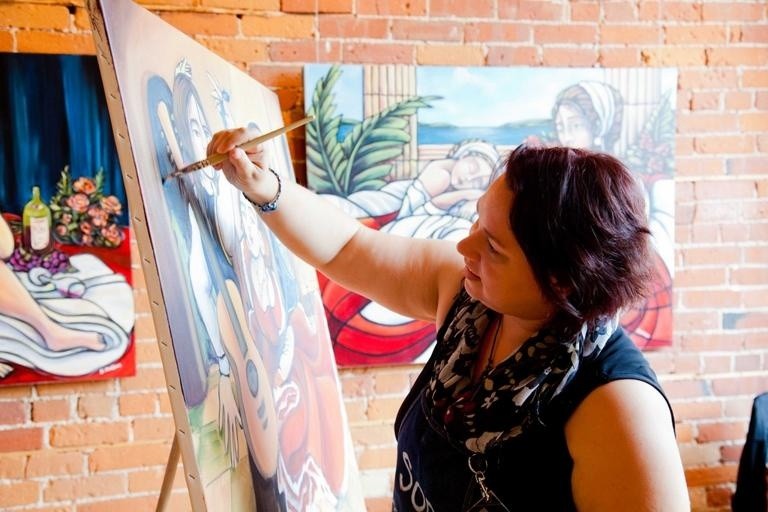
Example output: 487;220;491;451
171;60;252;474
206;127;691;512
145;76;198;246
0;216;109;352
552;84;625;152
376;139;504;245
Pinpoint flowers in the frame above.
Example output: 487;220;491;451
9;162;125;250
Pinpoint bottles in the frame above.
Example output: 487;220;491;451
22;186;54;255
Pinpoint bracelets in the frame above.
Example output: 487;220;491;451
242;168;282;212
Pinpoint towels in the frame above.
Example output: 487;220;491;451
0;253;135;377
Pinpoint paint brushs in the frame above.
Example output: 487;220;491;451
162;114;316;185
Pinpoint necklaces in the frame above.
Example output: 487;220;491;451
479;318;503;381
